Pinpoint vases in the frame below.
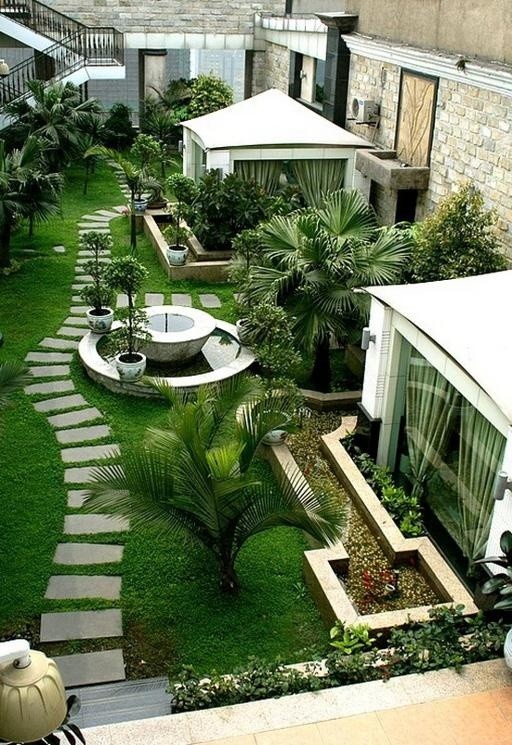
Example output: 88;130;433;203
257;410;291;446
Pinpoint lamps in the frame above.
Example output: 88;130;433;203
493;470;512;501
361;327;376;350
0;639;68;743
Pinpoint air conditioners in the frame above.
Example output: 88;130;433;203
350;96;375;122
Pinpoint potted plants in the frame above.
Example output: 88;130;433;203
130;134;161;214
79;231;152;383
162;172;202;266
